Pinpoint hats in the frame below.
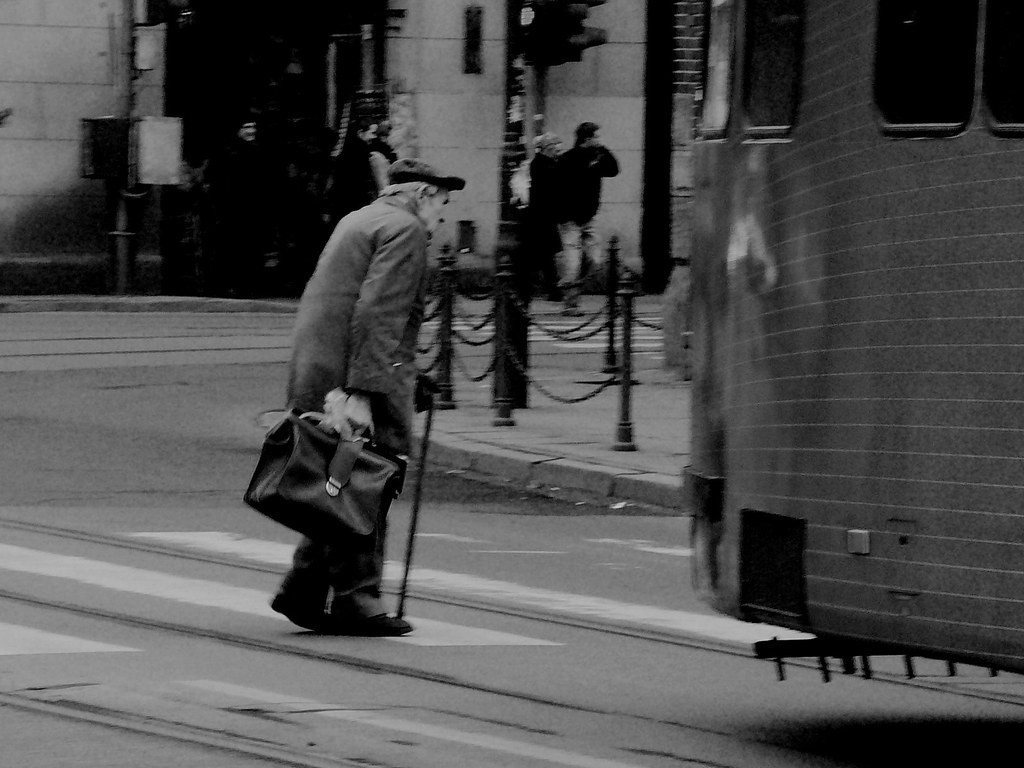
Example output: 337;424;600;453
533;130;562;152
387;157;466;191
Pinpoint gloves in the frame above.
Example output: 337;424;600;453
414;373;441;413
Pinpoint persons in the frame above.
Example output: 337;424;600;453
270;158;466;636
197;107;398;299
509;122;619;315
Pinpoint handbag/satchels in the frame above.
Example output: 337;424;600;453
244;407;400;563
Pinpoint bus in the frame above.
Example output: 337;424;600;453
683;0;1024;693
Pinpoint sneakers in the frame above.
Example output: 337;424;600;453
271;586;331;630
329;602;413;637
